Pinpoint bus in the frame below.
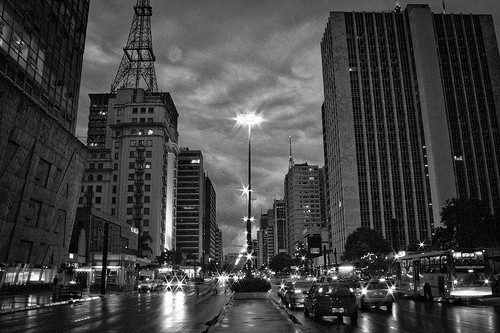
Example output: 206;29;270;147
398;246;493;305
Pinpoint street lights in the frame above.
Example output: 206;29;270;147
233;110;265;297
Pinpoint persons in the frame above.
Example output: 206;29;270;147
53;275;58;290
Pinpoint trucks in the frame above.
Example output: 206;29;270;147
326;265;354;281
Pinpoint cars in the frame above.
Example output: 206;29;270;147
136;280;159;292
352;276;392;311
167;275;187;290
304;279;359;325
305;273;317;283
284;278;317;308
137;268;155;287
